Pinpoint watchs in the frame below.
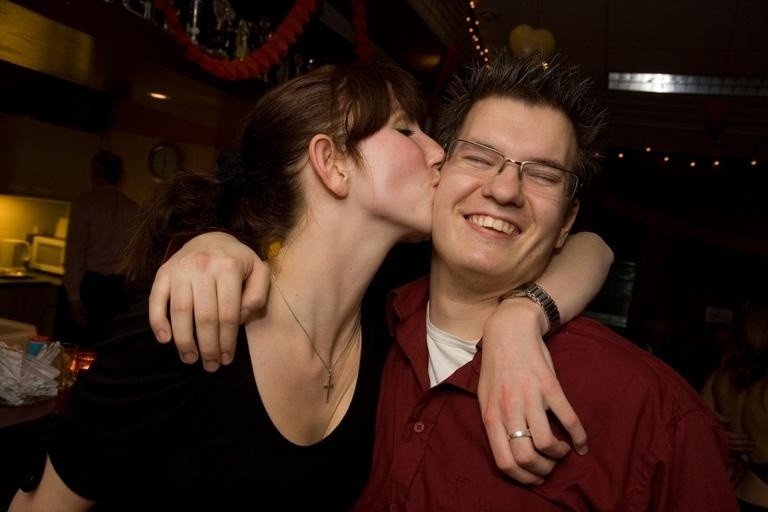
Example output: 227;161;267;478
499;282;561;338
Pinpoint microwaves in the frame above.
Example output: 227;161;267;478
29;235;66;277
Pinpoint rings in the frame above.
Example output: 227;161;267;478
506;429;531;440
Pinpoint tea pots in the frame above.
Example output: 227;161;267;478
0;236;31;278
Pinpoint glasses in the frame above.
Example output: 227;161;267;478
443;138;583;200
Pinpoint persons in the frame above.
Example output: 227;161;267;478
147;49;737;512
698;369;755;455
713;303;768;512
54;151;148;350
6;58;615;512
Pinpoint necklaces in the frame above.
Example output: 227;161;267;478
270;268;359;404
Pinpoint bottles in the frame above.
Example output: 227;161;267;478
28;336;51;354
